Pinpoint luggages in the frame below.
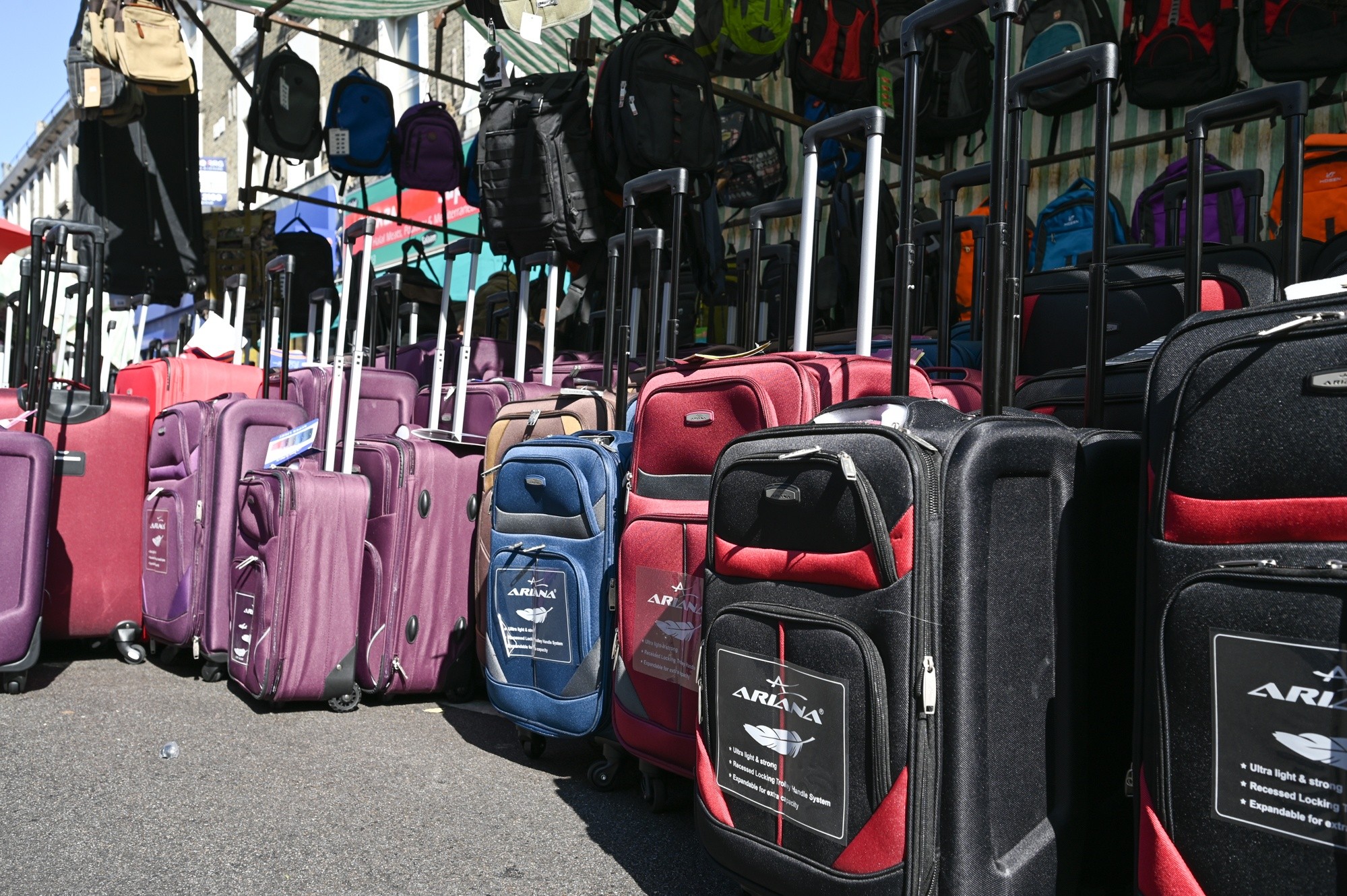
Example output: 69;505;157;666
0;42;1347;896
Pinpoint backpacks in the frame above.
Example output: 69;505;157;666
0;0;1347;381
